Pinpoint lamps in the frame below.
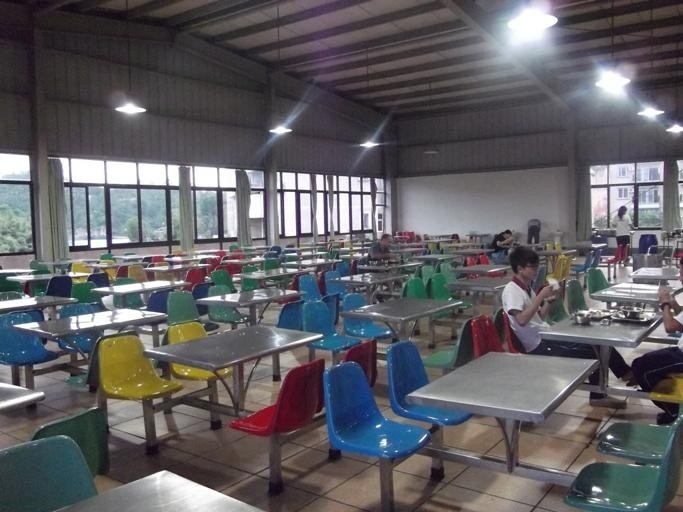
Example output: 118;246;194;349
600;0;629;97
640;0;666;118
422;0;443;154
116;0;146;117
667;0;683;135
358;0;380;149
267;0;294;137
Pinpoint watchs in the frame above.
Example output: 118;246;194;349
660;302;673;310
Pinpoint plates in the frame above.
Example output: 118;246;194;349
587;309;617;319
610;311;656;322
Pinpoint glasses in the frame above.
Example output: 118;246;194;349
521;263;539;269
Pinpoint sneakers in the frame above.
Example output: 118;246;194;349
657;410;676;426
622;370;634;381
589;396;629;409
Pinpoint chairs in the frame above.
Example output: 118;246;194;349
422;273;434;299
501;310;526;355
439;262;460;289
227;250;244;274
264;245;279;262
326;338;377;459
72;261;91;283
596;402;683;460
388;339;476;477
546;254;566;284
417;317;475;369
321;361;430;512
156;260;175;284
265;260;284;286
87;274;110;287
29;260;50;275
565;278;590;313
561;256;575;281
173;245;185;258
451;239;461;258
423;264;442;294
611;243;632;267
128;264;149;284
490;306;508;343
438;237;450;254
492;253;513;265
166;321;235;430
216;262;242;275
326;247;338;263
73;280;106;311
217;250;226;259
335;262;351;279
649;370;683;402
123;253;135;256
0;312;58;389
273;273;310;305
210;271;237;297
101;254;117;262
605;244;622;275
0;435;97;512
341;292;393;356
241;245;254;258
30;270;53;298
0;291;22;301
565;418;683;512
553;238;562;252
240;266;259;291
57;302;107;380
570;249;594;273
98;335;184;452
544;240;555;255
430;272;473;339
302;298;360;368
64;330;139;433
113;276;146;311
465;256;479;277
100;259;118;279
150;318;208;413
332;241;344;259
585;268;612;309
572;250;602;274
405;276;452;347
59;257;73;268
274;299;303;335
0;281;23;292
486;240;501;261
323;270;350;300
540;289;568;324
206;258;222;277
402;250;415;267
191;282;215;315
153;255;164;263
42;275;73;299
143;257;154;265
297;274;336;317
148;287;176;317
477;253;505;277
0;272;22;295
168;290;219;335
229;243;242;253
472;316;507;361
532;266;547;291
208;285;243;330
313;269;333;299
317;293;342;326
185;268;206;297
216;265;235;275
427;239;438;254
229;357;326;490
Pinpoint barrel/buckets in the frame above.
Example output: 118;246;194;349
638;234;659;254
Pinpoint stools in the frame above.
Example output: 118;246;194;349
399;278;409;297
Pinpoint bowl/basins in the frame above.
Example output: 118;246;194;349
620;306;642;319
369;260;378;266
574;313;590;325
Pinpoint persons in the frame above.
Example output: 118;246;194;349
501;245;639;409
367;233;402;305
491;228;514;260
632;257;683;426
612;205;638;266
527;219;541;244
